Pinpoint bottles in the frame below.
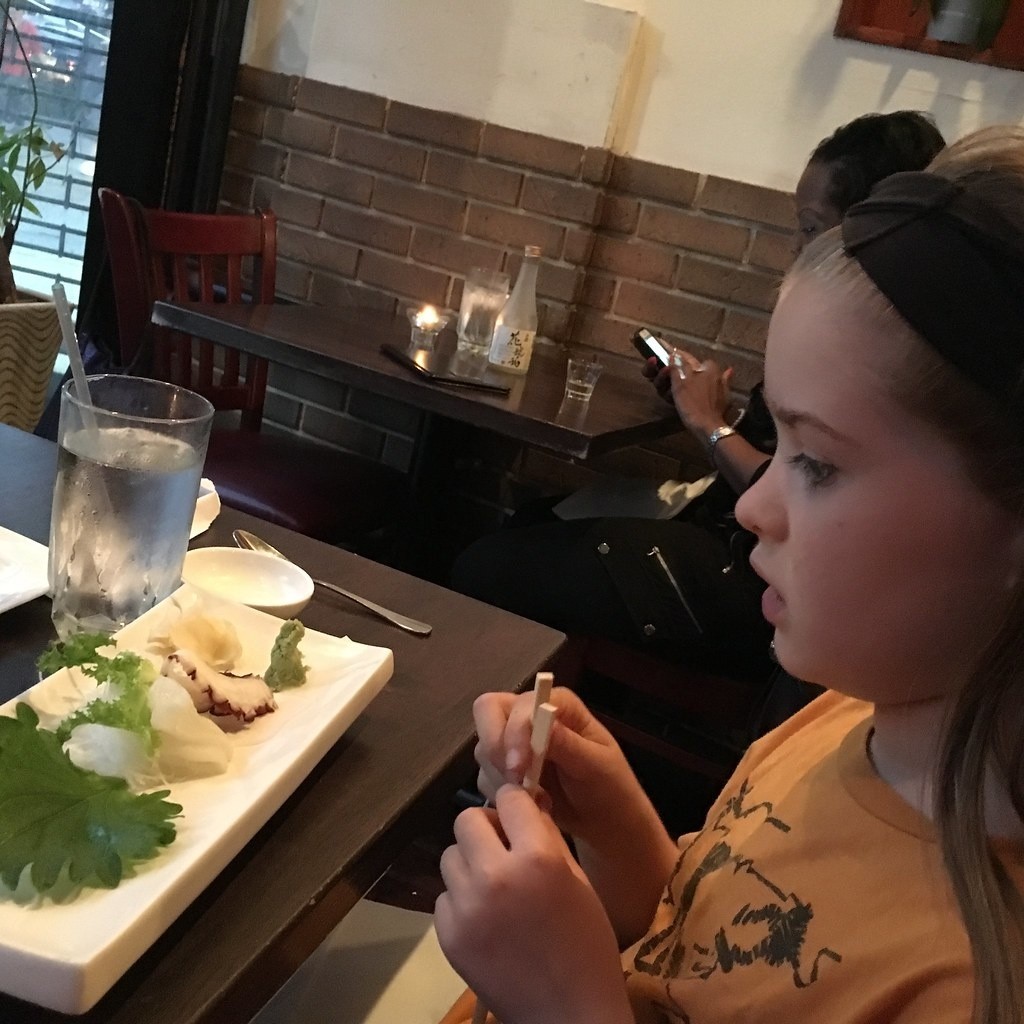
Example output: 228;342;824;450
487;245;540;375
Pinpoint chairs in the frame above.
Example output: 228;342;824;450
576;632;785;836
96;187;411;561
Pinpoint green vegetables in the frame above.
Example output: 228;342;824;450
0;628;182;892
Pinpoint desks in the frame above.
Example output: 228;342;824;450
151;299;687;609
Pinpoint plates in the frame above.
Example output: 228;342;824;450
0;524;51;616
0;583;394;1015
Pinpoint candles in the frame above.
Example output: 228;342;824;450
405;306;452;349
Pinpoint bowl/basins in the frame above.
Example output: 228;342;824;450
179;546;314;619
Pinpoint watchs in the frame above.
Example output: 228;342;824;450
707;425;735;456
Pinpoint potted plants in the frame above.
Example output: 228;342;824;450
0;2;77;438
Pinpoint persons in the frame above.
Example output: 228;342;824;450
434;124;1024;1024
450;108;946;662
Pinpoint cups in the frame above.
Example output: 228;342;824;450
52;374;215;648
456;267;509;345
565;359;603;402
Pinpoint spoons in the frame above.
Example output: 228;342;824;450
233;528;433;634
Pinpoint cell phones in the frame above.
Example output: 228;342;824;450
631;327;685;382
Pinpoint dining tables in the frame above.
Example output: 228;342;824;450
0;423;568;1024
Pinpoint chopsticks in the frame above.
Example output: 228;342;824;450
473;672;558;1024
